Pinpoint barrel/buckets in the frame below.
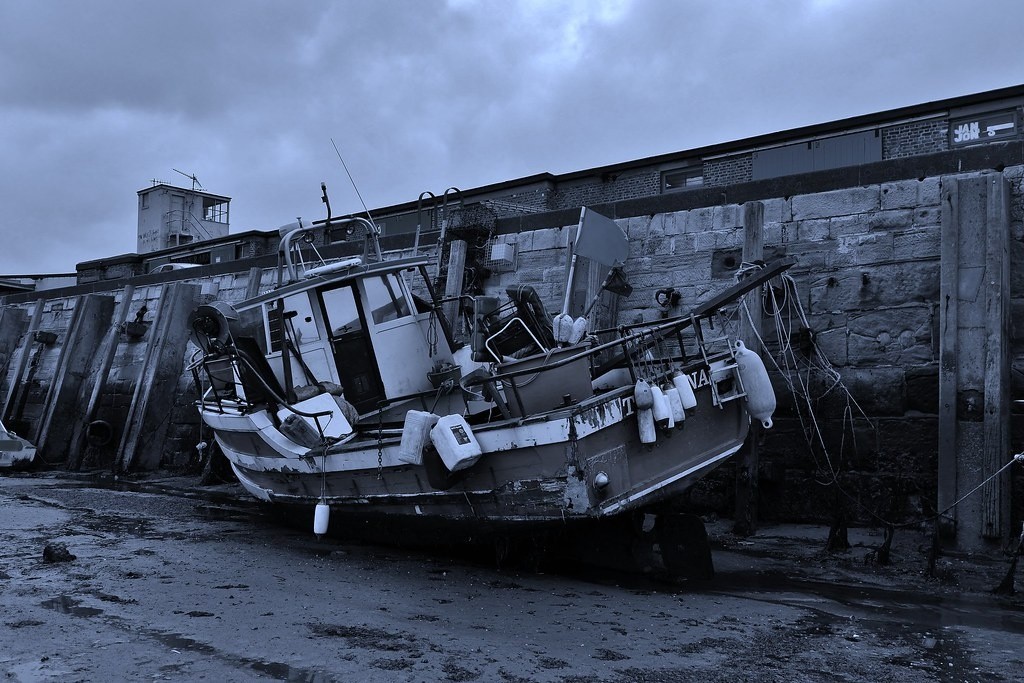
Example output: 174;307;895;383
430;414;482;472
397;409;441;466
279;414;323;449
499;343;594;418
453;344;507;414
423;441;466;491
428;365;461;388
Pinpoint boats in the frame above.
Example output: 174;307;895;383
183;138;754;554
0;421;37;473
